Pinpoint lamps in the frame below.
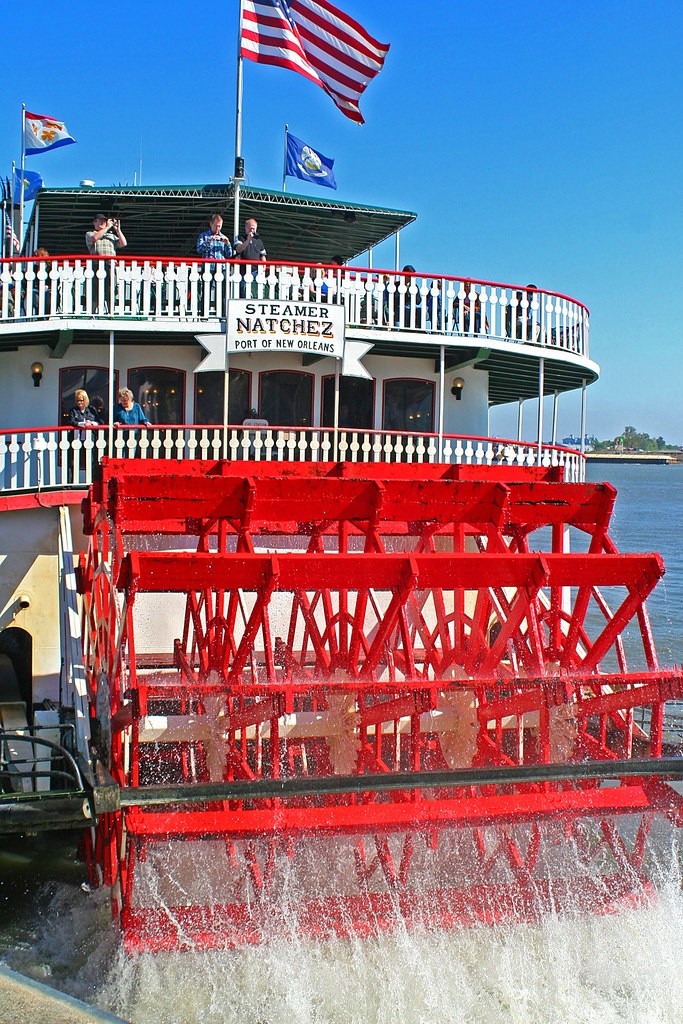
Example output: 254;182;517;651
451;376;465;402
31;362;45;387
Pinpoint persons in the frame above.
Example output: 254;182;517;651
326;255;345;274
0;261;25;322
21;247;61;321
453;278;481;337
310;262;337;304
506;291;540;345
233;219;267;298
85;214;127;320
196;213;232;323
426;280;448;334
68;387;104;490
106;387;152;458
364;273;390;331
387;265;421;332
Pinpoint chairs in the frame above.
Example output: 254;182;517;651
505;310;569;350
452;306;490;339
137;265;217;312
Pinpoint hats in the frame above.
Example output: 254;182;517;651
93;214;108;221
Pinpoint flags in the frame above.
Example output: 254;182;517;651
14;167;45;204
241;0;390;125
25;111;77;156
5;210;20;253
286;133;337;190
